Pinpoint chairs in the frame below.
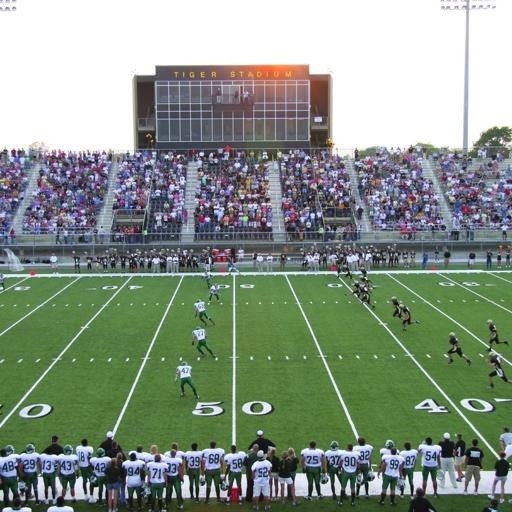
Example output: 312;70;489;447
277;143;363;248
354;144;512;247
195;144;274;247
0;143;188;246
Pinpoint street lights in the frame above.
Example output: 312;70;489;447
436;0;501;160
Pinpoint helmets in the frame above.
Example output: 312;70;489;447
397;479;406;490
91;476;97;486
386;440;393;448
356;473;364;484
221;482;228;490
330;441;338;449
97;448;105;457
26;444;34;453
366;472;375;481
321;476;328;484
437;469;444;479
63;445;72;454
5;445;13;454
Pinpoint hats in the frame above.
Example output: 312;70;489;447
257;430;263;435
444;433;450;439
107;431;112;437
52;437;57;442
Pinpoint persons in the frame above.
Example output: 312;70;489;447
235;87;239;104
215;88;221;103
146;133;152;149
243;90;248;105
